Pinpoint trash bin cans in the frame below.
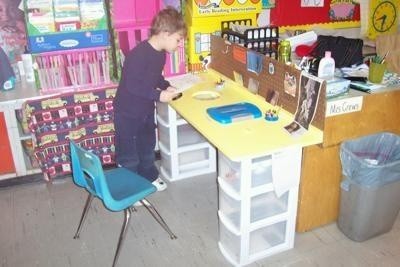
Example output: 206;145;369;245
338;132;400;242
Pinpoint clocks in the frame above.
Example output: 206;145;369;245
372;1;396;32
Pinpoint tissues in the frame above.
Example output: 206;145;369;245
323;77;351;99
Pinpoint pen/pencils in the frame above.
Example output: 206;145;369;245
369;48;391;64
36;50;110;90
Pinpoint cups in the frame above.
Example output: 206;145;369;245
369;60;387;83
36;60;114;94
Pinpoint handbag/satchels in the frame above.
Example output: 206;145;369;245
310;35;363;72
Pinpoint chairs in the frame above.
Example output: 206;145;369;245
69;140;177;266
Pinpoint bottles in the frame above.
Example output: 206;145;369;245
278;41;291;61
8;43;35;84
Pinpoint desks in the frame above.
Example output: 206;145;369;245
156;68;323;267
296;73;400;233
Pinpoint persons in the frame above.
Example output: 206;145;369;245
109;5;188;191
295;78;317;130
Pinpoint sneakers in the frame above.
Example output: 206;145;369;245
151;178;167;192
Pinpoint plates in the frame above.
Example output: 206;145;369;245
194;89;220;100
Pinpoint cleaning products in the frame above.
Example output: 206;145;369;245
20;45;35;82
